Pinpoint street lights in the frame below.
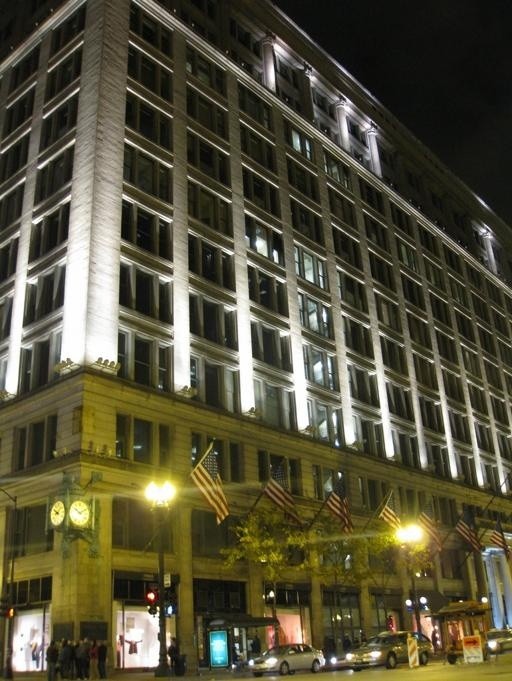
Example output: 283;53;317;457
0;488;17;681
143;477;179;678
393;523;426;640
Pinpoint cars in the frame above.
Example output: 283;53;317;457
484;629;511;655
330;641;364;672
248;644;327;677
344;631;434;670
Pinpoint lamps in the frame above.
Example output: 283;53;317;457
51;358;81;376
88;358;122;375
1;389;14;401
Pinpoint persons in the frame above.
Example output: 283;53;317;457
431;627;440;656
324;631;367;653
124;637;143;664
43;635;108;681
249;629;263;657
166;636;179;673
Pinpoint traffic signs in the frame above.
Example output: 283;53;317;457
164;572;171;587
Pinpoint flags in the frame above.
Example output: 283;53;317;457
378;491;408;532
325;478;359;535
454;506;485;553
186;441;231;528
414;502;445;551
489;515;512;561
264;458;306;527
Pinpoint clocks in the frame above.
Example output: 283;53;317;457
49;499;66;527
69;500;91;528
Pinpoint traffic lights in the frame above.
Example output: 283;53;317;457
7;607;16;619
147;586;157;615
0;593;13;619
